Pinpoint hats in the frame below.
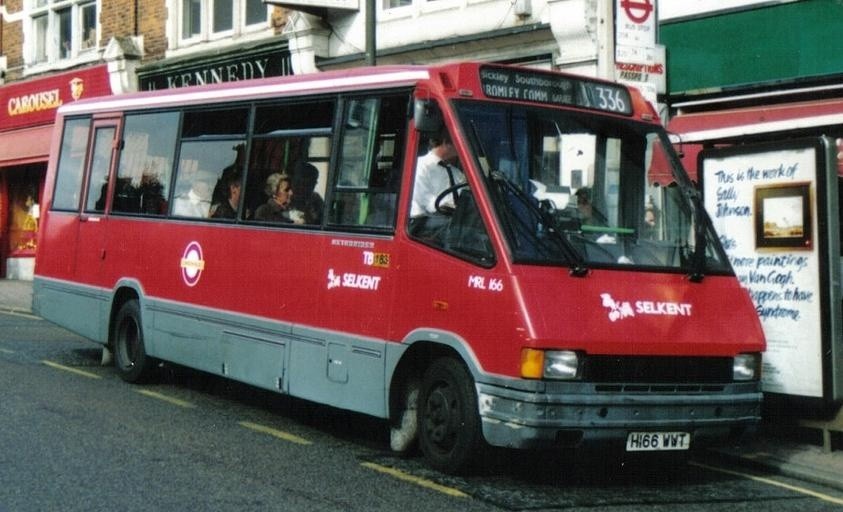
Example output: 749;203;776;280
264;173;288;195
195;170;217;181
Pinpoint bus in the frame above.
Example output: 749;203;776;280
29;64;766;477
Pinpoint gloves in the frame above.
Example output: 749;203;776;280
288;209;306;225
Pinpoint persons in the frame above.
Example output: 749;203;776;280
409;124;470;220
644;207;657;239
72;142;328;224
19;194;40;250
576;188;596;227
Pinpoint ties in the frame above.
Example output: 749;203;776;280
438;159;459;206
